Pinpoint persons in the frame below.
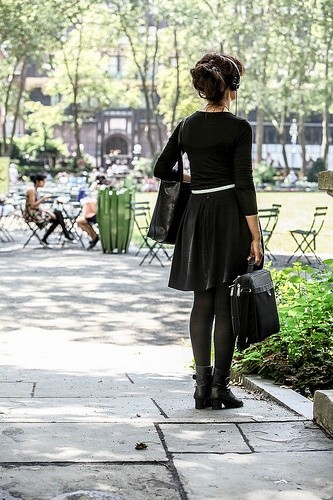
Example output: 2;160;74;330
76;176;107;250
154;53;264;410
24;174;75;248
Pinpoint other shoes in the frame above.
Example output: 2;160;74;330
86;233;99;251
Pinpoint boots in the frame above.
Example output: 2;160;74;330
193;365;212;409
209;367;243;410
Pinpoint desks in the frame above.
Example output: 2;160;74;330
58;202;82;245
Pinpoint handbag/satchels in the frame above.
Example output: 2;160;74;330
228;255;281;352
147;117;192;245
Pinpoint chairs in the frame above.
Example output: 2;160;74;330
286;206;328;265
0;200;282;268
